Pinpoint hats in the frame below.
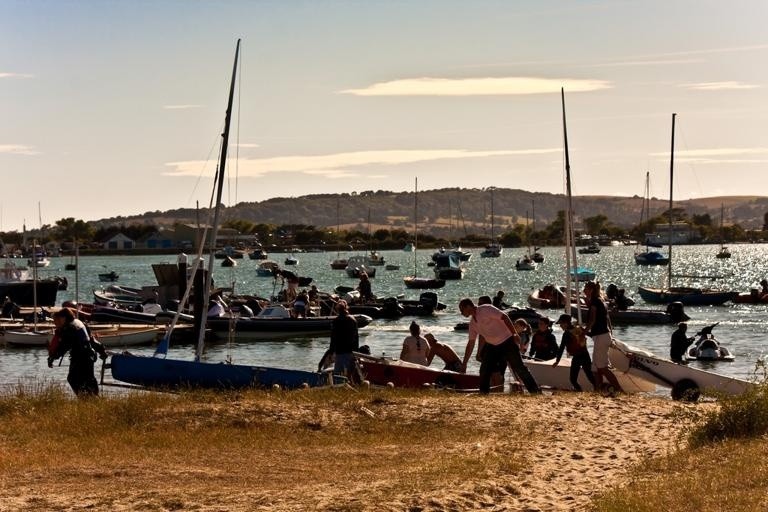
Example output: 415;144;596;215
555;314;571;324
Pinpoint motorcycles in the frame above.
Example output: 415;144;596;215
684;319;737;363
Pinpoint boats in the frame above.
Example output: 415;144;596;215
575;231;664;256
0;176;474;355
607;335;761;405
484;243;503;252
570;266;598;281
715;200;733;259
571;301;693;326
354;350;480;394
526;281;586;309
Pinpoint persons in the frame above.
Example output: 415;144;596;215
46;307;107;397
281;279;319;316
50;313;108;361
319;343;371;373
460;281;626;396
357;273;371;300
670;323;696;365
334;299;359;377
424;332;465;372
401;321;430;366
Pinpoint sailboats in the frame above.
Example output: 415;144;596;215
633;171;669;267
516;85;654;394
639;112;768;306
530;199;545;263
401;177;446;290
480;186;502;258
515;210;539;270
95;34;352;393
